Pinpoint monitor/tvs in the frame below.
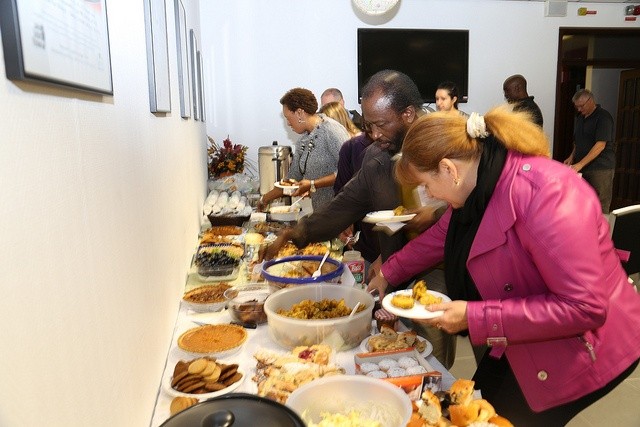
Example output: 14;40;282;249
358;28;467;103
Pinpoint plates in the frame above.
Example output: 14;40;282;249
382;289;453;319
274;182;300;189
195;242;247;259
199;225;249;237
366;210;417;222
361;332;434;359
164;360;246;398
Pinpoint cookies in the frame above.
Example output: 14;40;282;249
171;354;242;395
169;396;198;417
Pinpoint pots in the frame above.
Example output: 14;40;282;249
159;393;308;427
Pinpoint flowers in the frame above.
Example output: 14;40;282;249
207;136;250;180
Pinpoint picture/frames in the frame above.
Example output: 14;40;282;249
196;49;207;123
144;0;172;114
0;1;114;95
174;0;191;119
190;29;200;121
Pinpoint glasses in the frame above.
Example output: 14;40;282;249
579;97;590;109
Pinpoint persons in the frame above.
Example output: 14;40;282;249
502;74;544;133
254;87;351;228
433;79;473;122
320;88;362;124
254;68;458;372
319;101;360;137
562;87;617;218
363;109;640;427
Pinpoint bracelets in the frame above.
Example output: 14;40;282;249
259;195;265;206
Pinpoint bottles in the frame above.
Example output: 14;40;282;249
375;308;399;333
342;250;365;288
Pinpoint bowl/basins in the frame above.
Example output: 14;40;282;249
223;283;283;324
262;255;344;293
182;284;239;313
284;375;413;427
264;283;375;352
269;206;299;221
194;254;246;282
208;209;251;227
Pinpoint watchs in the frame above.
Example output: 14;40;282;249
309;179;317;193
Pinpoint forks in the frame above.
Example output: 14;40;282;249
251;259;266;282
312;250;330;277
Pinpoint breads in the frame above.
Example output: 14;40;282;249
408;379;513;426
360;356;429;381
369;323;427;353
388;279;444;310
252;343;346;407
